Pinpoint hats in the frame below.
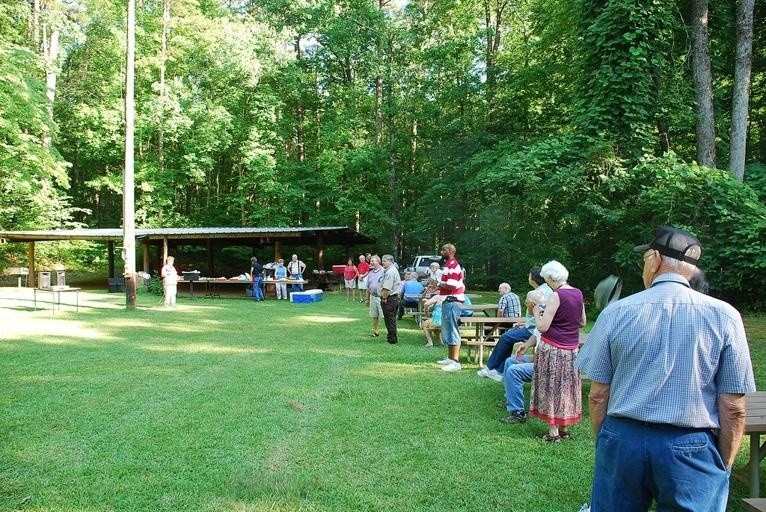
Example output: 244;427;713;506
635;224;704;266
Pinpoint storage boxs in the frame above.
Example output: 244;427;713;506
305;289;323;303
184;273;200;281
332;265;346;275
290;292;311;304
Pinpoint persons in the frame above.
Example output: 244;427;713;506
343;243;474;372
496;290;546;425
161;255;182;309
477;266;554;383
274;258;288;300
485;282;522;341
532;260;587;443
287;253;306;292
571;225;757;511
250;256;265;302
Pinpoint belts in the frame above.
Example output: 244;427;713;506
444;298;465;302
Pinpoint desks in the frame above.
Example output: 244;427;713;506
463;303;499;317
731;392;766;499
158;279;305;304
311;271;344;291
404;294;482;330
34;286;81;317
459;317;535;364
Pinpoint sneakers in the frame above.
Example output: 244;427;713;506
536;432;560;443
256;299;259;302
497;399;508;408
368;332;379;337
500;413;527;423
558;429;570;439
259;298;265;301
482;369;504;383
436;357;451;364
477;367;490;378
442;362;461;371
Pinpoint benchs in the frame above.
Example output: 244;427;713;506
483;325;507;335
410;311;430;329
742;498;766;512
466;341;499;369
461;335;501;361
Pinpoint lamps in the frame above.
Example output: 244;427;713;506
266;237;272;244
259;238;265;245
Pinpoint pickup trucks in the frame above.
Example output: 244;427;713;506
400;255;466;287
